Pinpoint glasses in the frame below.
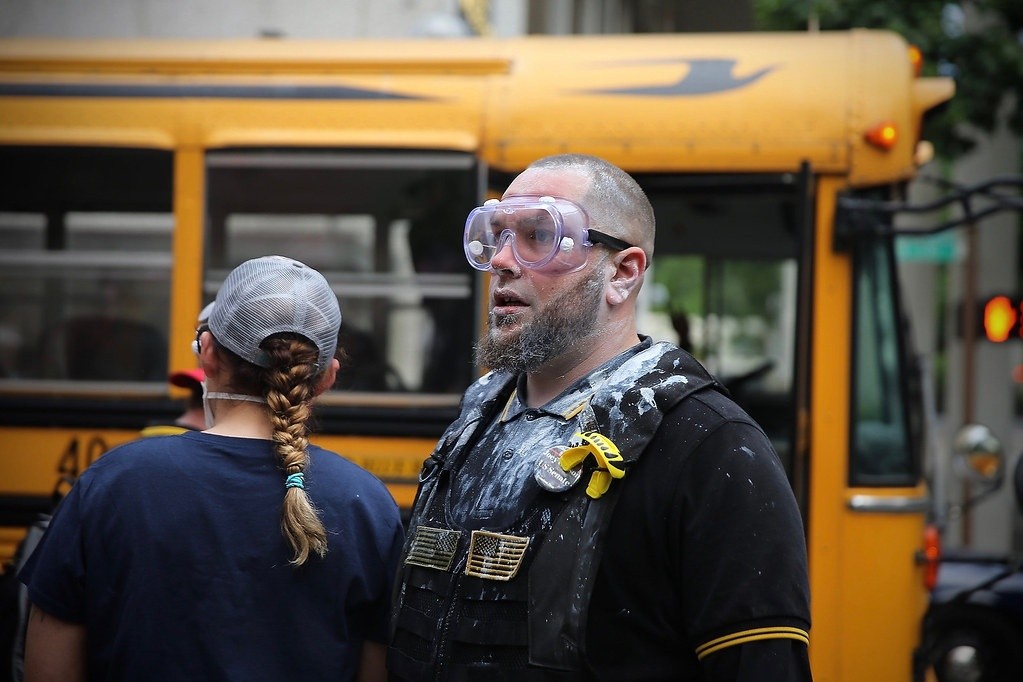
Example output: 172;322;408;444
463;196;591;276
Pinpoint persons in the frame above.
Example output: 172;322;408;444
24;254;409;682
387;152;814;682
137;367;209;437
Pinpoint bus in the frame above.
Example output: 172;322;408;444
0;28;1023;682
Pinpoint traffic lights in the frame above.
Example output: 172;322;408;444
965;294;1022;341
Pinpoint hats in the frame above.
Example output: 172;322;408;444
197;255;341;377
171;368;207;389
